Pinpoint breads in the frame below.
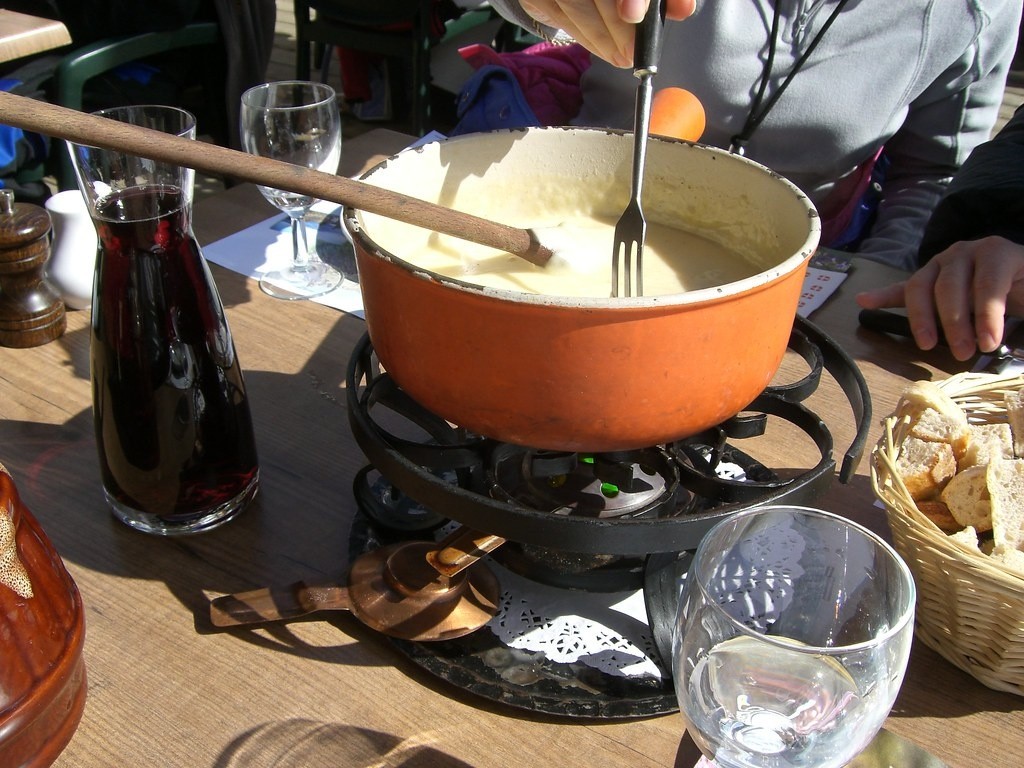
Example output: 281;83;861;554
891;381;1024;577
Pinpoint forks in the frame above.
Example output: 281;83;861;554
612;1;667;301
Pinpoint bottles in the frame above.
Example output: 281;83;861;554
41;190;102;310
66;106;261;537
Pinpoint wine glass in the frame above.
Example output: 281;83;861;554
240;81;342;300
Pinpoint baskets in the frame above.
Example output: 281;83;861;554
870;372;1024;696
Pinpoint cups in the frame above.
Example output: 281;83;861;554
673;506;916;768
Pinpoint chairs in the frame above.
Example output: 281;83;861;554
15;21;224;206
290;0;444;139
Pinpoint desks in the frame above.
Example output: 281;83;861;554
0;129;1024;768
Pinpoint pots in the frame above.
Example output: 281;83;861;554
345;127;822;450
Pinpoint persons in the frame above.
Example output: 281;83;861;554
450;0;1023;270
852;98;1023;365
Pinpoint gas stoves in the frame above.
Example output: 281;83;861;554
209;308;875;643
